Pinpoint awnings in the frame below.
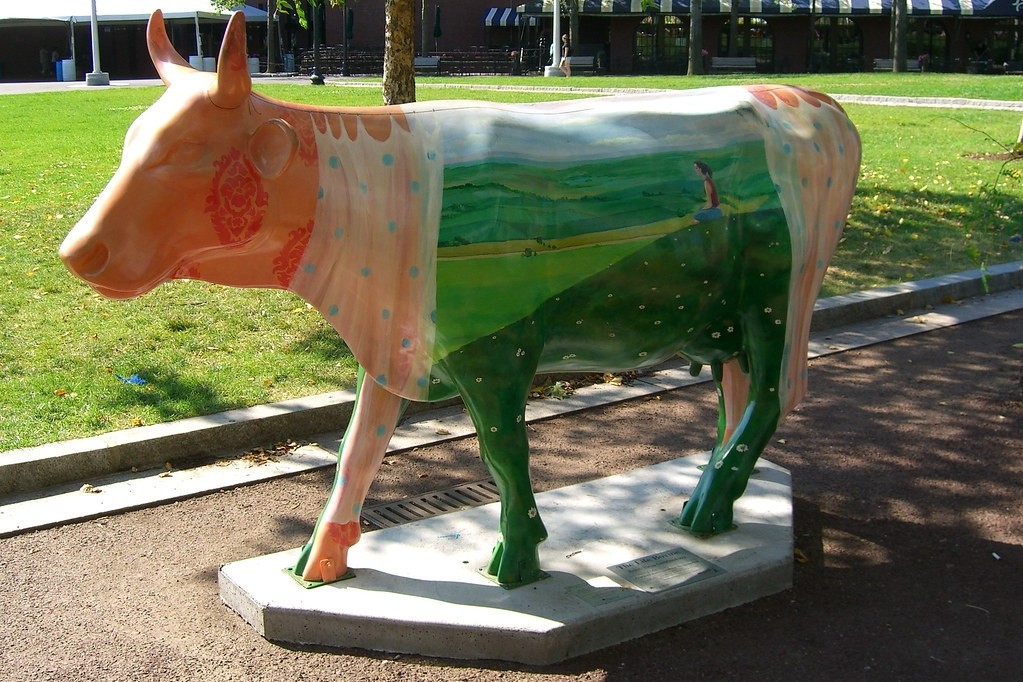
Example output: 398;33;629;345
482;7;540;27
512;0;1023;19
0;0;280;61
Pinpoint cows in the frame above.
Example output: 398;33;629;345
57;9;864;584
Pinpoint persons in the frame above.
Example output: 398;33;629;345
37;45;53;78
559;34;572;77
51;47;60;64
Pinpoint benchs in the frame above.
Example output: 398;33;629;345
564;56;597;76
711;58;757;74
873;59;921;71
414;56;441;77
